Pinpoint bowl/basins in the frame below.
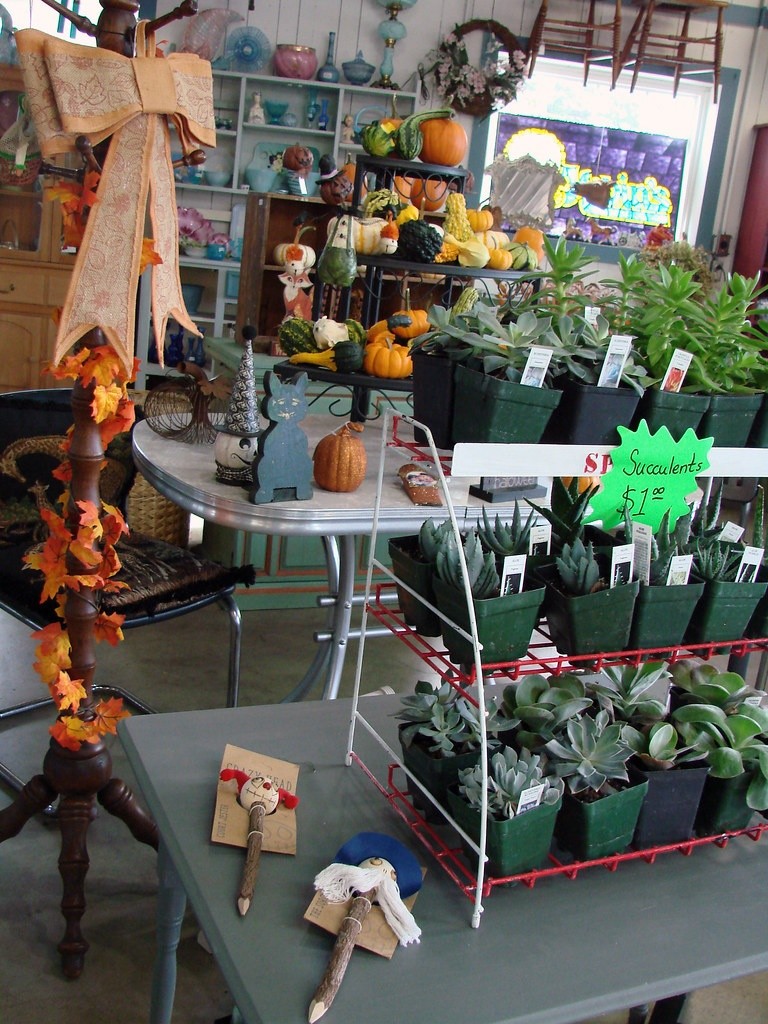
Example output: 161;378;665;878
203;170;230;187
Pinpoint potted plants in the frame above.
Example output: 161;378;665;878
386;662;768;878
406;233;768;450
387;477;768;665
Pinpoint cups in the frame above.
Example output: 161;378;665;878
207;243;227;260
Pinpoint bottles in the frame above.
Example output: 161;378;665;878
304;88;320;129
148;322;206;368
317;31;340;83
318;100;329;131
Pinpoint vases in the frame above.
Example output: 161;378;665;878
317;32;340;82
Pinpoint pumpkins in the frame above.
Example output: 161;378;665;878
273;94;545;379
313;423;367;492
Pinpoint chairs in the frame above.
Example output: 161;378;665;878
0;388;242;818
523;0;729;103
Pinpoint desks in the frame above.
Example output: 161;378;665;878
132;413;626;701
116;674;768;1024
312;252;543;330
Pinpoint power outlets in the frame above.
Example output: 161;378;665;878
711;259;723;280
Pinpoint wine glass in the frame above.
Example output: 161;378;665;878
264;99;289;125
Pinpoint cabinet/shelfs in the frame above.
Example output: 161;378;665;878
339;156;466;420
234;192;474;353
345;408;768;929
134;69;422;390
0;62;113;393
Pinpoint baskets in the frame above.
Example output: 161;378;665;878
0;151;42;186
123;474;190;548
